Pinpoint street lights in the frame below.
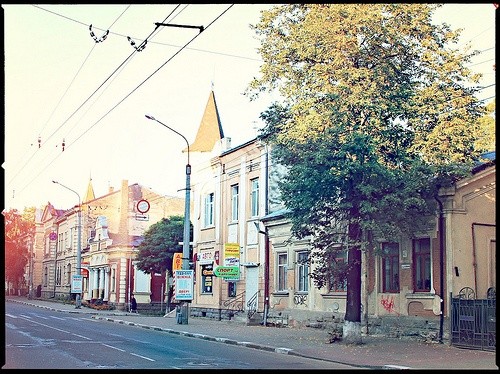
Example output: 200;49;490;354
144;112;193;325
52;180;85;310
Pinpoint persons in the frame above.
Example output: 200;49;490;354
130;294;137;310
